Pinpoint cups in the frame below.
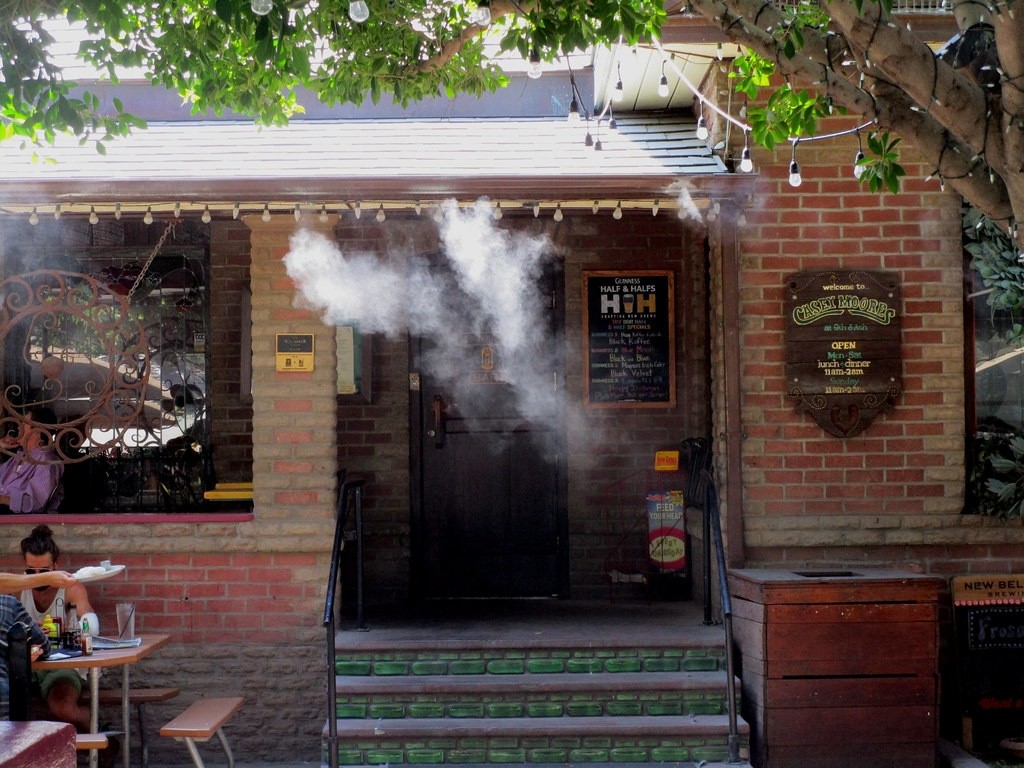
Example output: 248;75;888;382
68;630;82;650
115;603;135;640
50;617;63;642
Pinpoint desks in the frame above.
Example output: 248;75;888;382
32;634;170;768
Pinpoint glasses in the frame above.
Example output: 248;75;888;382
25;567;52;575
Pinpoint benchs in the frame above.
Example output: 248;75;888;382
161;697;246;768
81;688;180;768
76;734;108;748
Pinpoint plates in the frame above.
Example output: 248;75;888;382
69;564;126;583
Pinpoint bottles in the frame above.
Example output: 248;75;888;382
43;615;57;649
82;618;93;656
66;604;79;649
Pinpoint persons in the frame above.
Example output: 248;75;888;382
0;569;79;595
0;408;64;515
0;593;52;720
10;524;122;768
